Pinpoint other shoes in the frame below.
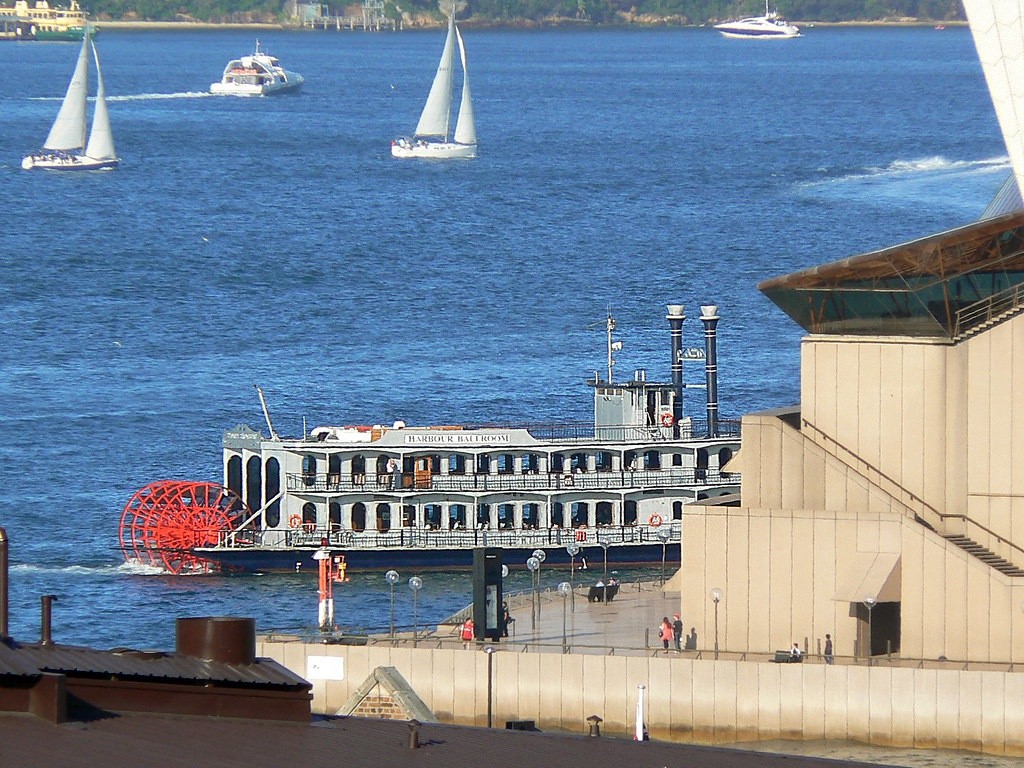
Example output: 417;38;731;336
674;651;680;654
663;651;668;653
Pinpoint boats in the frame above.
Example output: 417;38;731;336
713;13;801;40
0;0;100;40
208;53;304;97
188;304;742;560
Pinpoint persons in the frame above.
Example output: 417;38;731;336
659;617;674;653
824;634;832;665
789;643;801;658
460;617;474;650
671;613;682;654
594;577;617;602
502;601;509;637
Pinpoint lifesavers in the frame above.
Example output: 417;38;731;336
576;531;586;541
288;514;302;528
649;513;661;526
564;475;574;486
303;520;316;533
661;413;675;427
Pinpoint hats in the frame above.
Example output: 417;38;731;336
673;611;680;617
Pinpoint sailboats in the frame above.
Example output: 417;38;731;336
392;6;478;160
20;28;113;171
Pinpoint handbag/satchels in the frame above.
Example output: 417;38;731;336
659;628;663;637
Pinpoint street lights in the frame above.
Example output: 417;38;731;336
598;537;611;578
557;581;571;656
710;587;723;658
385;569;398;645
525;557;535;635
658;529;668;585
863;592;875;666
566;543;578;607
485;643;494;731
532;549;547;624
409;575;420;649
